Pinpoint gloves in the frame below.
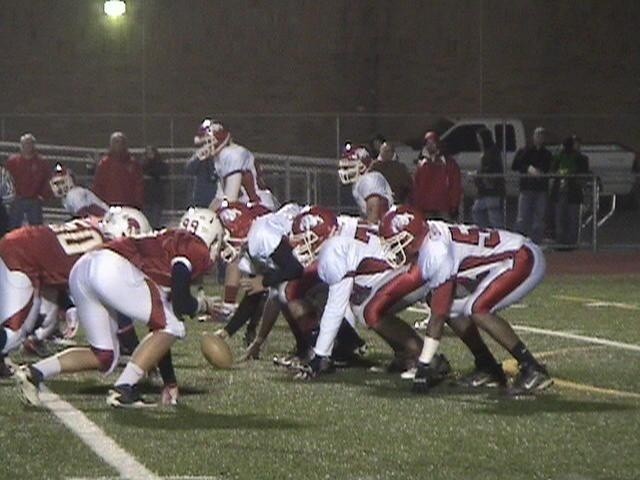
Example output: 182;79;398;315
292;356;333;382
412;361;431;394
193;291;230;320
161;378;181;409
59;307;80;340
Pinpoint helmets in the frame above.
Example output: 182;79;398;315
334;143;372;186
288;203;338;273
48;165;76;199
101;205;154;241
212;200;255;266
376;204;430;272
193;120;231;163
179;206;223;267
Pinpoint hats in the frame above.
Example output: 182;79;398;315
424;130;439;143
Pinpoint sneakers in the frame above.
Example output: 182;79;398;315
272;346;303;368
48;335;77;348
26;335;50;357
334;342;370;368
0;356;14;385
12;361;47;414
507;367;554;401
106;384;159;412
431;355;453;382
451;365;505;391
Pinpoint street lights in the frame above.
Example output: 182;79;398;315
104;0;147;150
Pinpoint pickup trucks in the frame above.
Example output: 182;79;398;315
388;115;635;221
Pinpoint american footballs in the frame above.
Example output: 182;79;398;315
200;331;233;368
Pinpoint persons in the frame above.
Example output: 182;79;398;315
93;132;143;209
0;168;15;232
217;202;369;366
334;145;396;224
142;145;168;225
242;286;282;364
370;143;411;207
193;119;281;213
2;134;53;230
512;126;552;247
184;153;217;206
14;208;223;410
551;136;589;248
413;131;462;222
471;128;506;228
290;206;428;383
0;205;153;384
379;204;555;398
49;167;107;217
